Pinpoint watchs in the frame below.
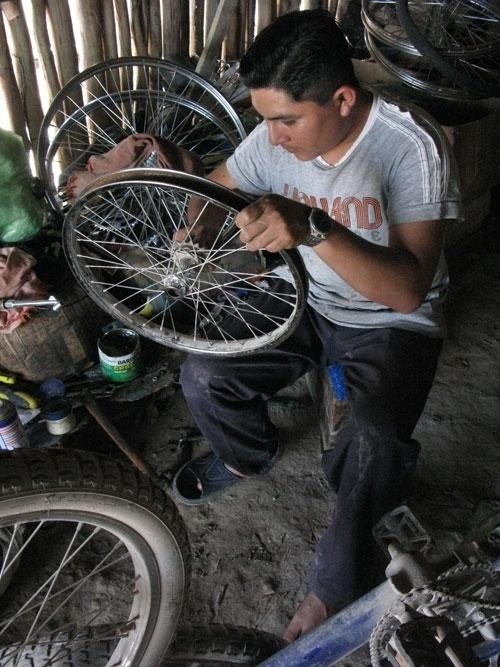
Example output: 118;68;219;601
302;207;335;248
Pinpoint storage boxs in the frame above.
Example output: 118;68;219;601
0;289;98;381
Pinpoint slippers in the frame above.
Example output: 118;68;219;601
172;424;280;506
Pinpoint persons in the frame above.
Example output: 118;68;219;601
170;7;465;644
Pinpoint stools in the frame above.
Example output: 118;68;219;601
304;365;351;452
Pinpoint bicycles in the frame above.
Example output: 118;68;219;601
0;446;500;667
